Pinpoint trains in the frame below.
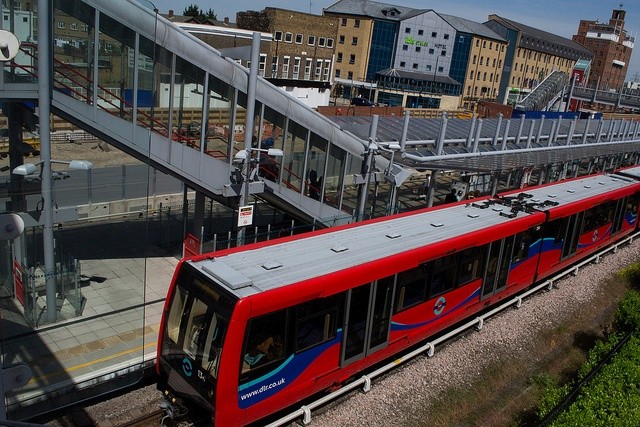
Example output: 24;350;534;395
156;164;640;427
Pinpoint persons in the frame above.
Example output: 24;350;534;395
316;175;326;203
584;211;608;231
465;190;477;200
456;190;466;202
474;190;482;197
444;188;458;203
335;188;341;209
250;135;280;183
422;173;431;195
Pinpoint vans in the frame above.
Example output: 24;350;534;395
352;98;373;106
